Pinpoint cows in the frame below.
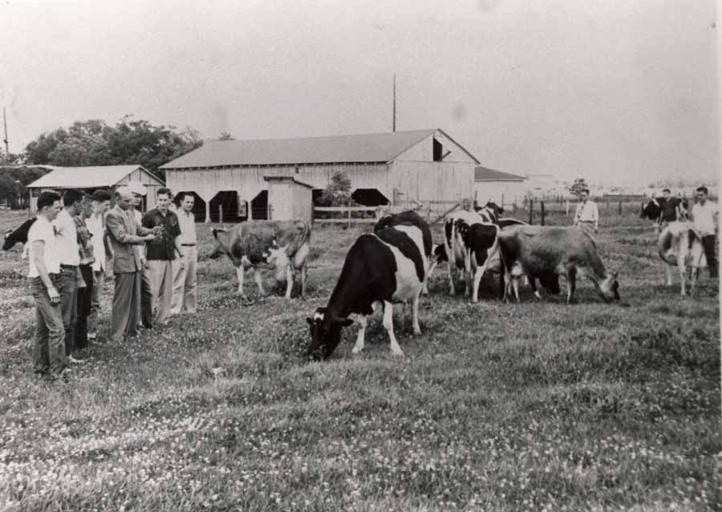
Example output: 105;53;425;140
367;206;432;297
305;223;430;361
498;223;620;306
640;197;666;225
435;201;559;305
3;218;39;258
206;218;315;302
652;220;706;299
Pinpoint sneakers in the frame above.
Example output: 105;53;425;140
33;336;111;383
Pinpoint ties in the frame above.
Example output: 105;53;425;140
577;205;585;218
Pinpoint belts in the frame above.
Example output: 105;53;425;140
578;220;594;224
181;244;196;246
60;263;79;270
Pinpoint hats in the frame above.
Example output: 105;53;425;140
126;182;147;196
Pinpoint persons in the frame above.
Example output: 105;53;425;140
26;191;73;381
690;186;721;279
655;188;684;233
127;180;163;332
103;185;168;344
170;192;201;318
52;188;85;363
72;194;94;350
574;189;600;236
141;187;186;328
82;189;110;339
